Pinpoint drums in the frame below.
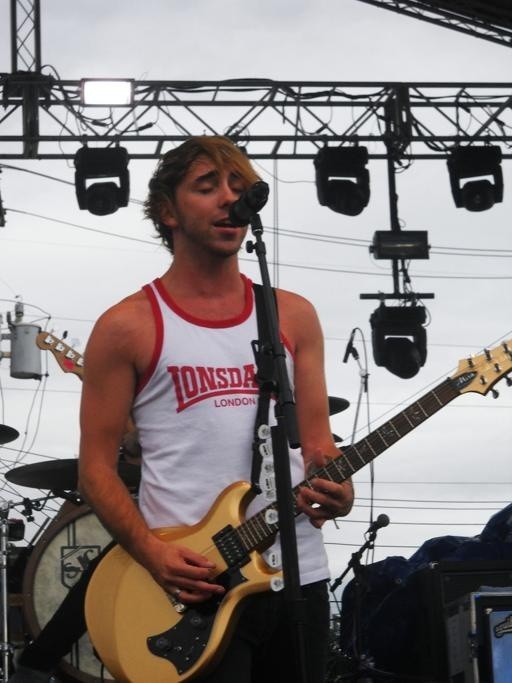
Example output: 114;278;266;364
22;503;114;683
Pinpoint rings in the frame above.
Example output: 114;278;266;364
174;591;180;595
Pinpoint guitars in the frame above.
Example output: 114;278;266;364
35;325;83;381
80;333;511;682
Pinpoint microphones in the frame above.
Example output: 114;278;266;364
367;513;390;534
229;181;269;227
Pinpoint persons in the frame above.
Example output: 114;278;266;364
77;136;355;682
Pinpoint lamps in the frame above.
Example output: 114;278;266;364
80;77;134;108
0;324;43;382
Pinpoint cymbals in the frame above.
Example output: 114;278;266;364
0;424;19;446
3;457;139;491
328;393;349;416
